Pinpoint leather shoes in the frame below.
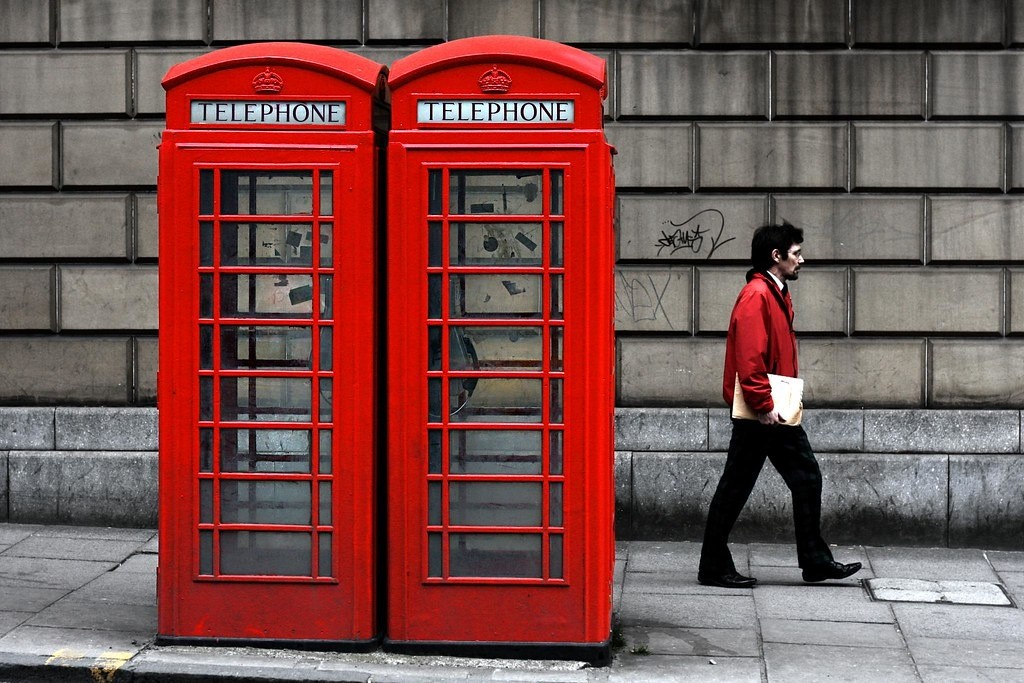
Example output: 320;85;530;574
802;558;863;583
698;568;758;588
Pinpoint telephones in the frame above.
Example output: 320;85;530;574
427;276;480;400
306;277;332;391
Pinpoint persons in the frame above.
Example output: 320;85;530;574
695;219;863;588
